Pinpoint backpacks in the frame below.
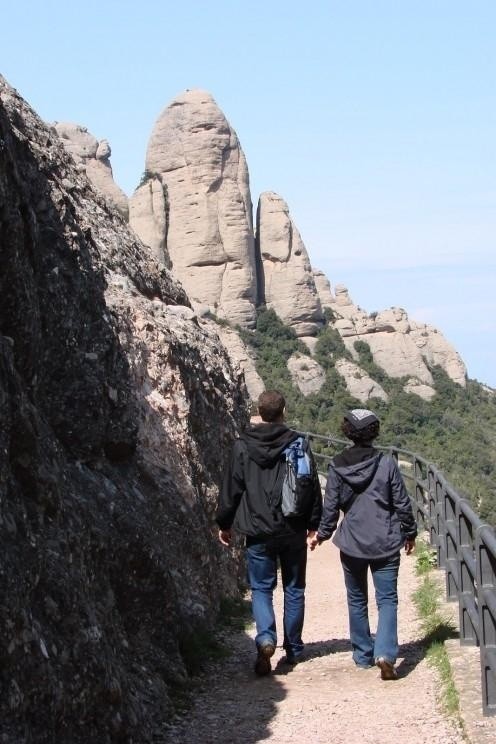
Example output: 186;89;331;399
280;437;321;516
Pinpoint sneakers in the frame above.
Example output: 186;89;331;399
375;657;397;680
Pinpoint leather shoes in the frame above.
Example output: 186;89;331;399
255;645;275;676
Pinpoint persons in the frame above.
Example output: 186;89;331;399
217;390;323;677
306;408;419;681
